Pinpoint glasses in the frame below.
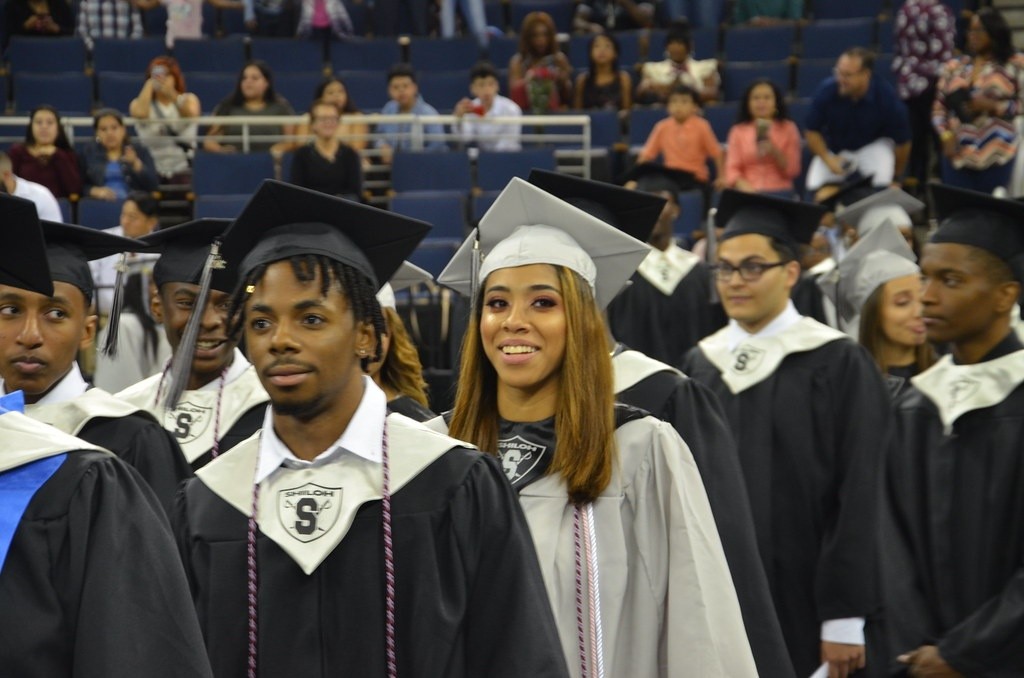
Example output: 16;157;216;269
704;257;792;283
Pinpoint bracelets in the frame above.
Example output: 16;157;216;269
174;96;182;106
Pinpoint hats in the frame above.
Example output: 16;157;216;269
437;175;651;316
100;217;239;360
832;185;926;244
710;187;828;259
527;163;668;245
815;217;920;324
0;193;55;299
375;260;434;316
923;177;1024;281
622;167;697;198
37;216;150;360
159;178;435;409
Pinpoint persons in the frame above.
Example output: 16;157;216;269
678;188;892;678
0;0;1024;393
167;178;569;678
815;217;938;391
366;259;439;423
529;170;795;678
112;217;270;469
607;161;713;372
0;193;211;678
415;178;760;678
874;183;1024;678
0;219;191;525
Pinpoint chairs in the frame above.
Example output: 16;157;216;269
0;0;1023;308
